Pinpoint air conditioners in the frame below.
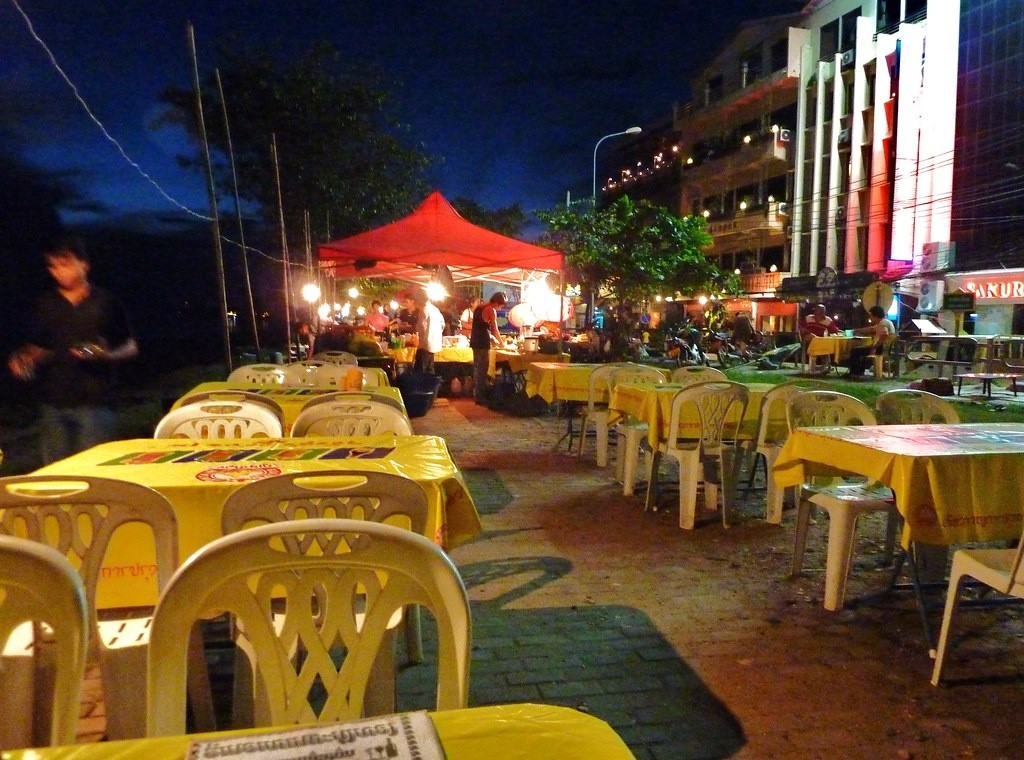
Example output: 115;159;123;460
918;280;945;312
780;129;792;141
785;226;792;239
920;242;956;273
838;128;851;144
777;202;791;216
842;49;854;66
835;206;846;219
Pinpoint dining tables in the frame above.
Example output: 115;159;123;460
490;349;571;413
772;423;1024;658
808;335;873;374
171;382;415;436
366;368;391;387
525;361;672;451
0;436;484;663
611;383;816;508
383;347;473;362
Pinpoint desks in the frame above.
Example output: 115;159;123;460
953;373;1024;398
0;703;635;760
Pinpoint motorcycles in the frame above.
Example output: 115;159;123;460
711;330;749;368
672;322;710;368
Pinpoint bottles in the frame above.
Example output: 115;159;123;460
415;331;419;346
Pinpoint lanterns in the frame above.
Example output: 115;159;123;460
531;294;575;332
507;301;545;337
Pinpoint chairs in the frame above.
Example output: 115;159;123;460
22;351;471;748
859;333;898;380
576;362;1024;687
799;328;831;374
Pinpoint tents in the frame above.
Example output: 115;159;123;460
315;190;565;364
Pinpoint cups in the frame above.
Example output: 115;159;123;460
845;329;853;337
823;329;828;336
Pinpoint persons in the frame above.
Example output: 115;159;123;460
7;234;141;467
845;305;896;380
792;304;840;374
468;290;509;405
638;328;652;344
459;295;482;340
293;292;420;358
440;298;458;335
413;290;446;374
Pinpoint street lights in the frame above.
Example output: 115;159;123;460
585;125;644;321
